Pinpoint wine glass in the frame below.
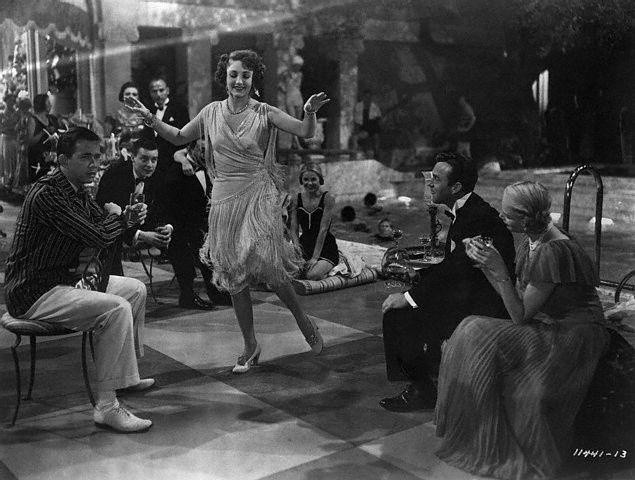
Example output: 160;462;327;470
418;232;433;262
390;230;404;260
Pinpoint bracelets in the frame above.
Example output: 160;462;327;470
306;112;315;116
496;277;510;283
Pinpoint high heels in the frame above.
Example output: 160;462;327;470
305;315;323;355
233;343;261;374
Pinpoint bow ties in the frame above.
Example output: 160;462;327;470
135;176;149;185
153;106;163;111
76;189;90;199
444;209;455;219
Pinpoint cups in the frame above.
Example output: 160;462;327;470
128;191;146;225
476;234;495;248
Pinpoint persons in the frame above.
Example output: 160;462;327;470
354;90;383;155
455;94;475;158
372;218;403;242
285;161;340;281
2;128;155;431
431;180;609;479
121;50;330;374
379;154;517;411
354;193;387;213
332;204;366;226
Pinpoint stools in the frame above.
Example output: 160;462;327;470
1;307;99;425
131;235;197;303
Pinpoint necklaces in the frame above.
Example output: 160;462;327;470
529;224;554;251
227;98;250;114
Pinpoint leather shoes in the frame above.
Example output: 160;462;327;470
382;385;435;413
116;379;154;394
91;401;151;434
178;292;212;311
